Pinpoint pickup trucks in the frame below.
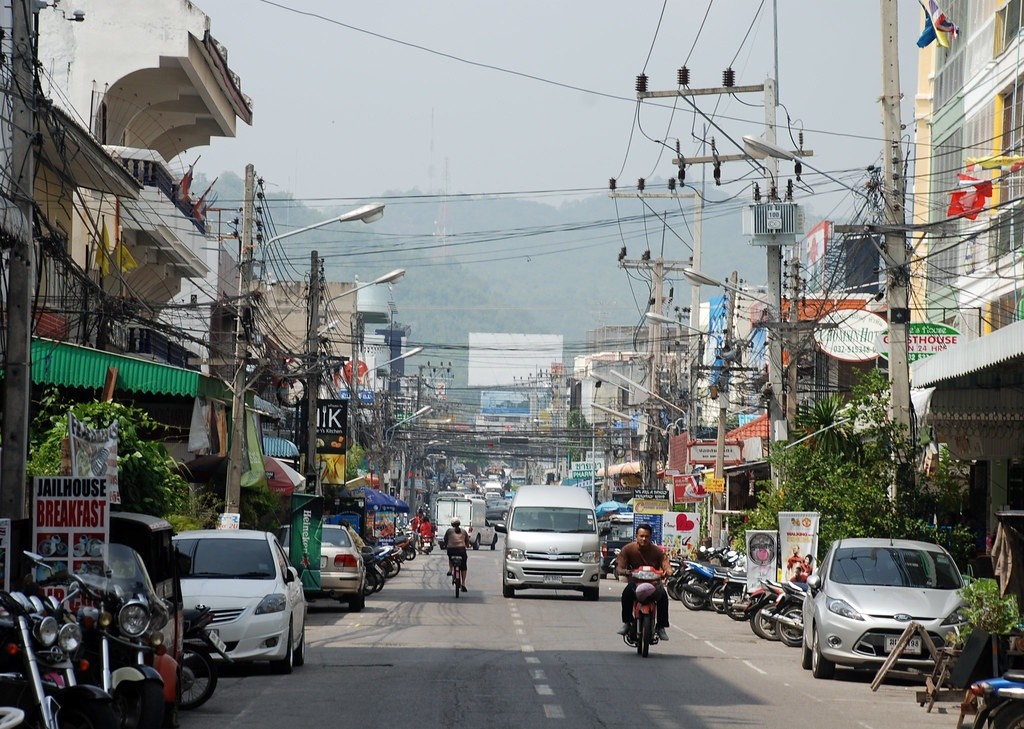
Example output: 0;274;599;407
436;496;499;549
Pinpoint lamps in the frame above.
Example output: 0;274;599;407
53;5;84;22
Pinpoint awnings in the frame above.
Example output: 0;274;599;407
31;338;300;457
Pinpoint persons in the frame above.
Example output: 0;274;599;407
616;524;671;640
412;510;470;592
785;546;813;585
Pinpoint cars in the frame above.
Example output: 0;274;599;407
278;524;373;612
802;536;965;677
426;453;525;520
172;531;304;665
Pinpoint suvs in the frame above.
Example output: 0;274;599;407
600;519;633;578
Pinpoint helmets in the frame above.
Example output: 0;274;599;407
451;517;460;524
635;582;656;601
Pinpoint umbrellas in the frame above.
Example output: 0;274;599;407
351;485;409;538
598;501;627;517
262;458;306;495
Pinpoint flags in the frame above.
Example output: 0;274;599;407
947;154;1024;221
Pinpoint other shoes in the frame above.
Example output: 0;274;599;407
461;585;467;592
616;622;631;635
655;623;669;640
447;572;452;576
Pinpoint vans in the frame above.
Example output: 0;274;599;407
494;485;601;602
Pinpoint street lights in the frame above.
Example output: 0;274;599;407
591;268;789;500
225;197;386;519
302;266;434;500
739;135;918;496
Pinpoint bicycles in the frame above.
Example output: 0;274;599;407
446;545;462;599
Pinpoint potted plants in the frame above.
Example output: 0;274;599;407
945;577;1024;690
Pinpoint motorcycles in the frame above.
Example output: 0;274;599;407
967;668;1024;728
665;545;751;620
618;566;668;657
745;579;810;646
360;530;433;596
0;542;234;729
